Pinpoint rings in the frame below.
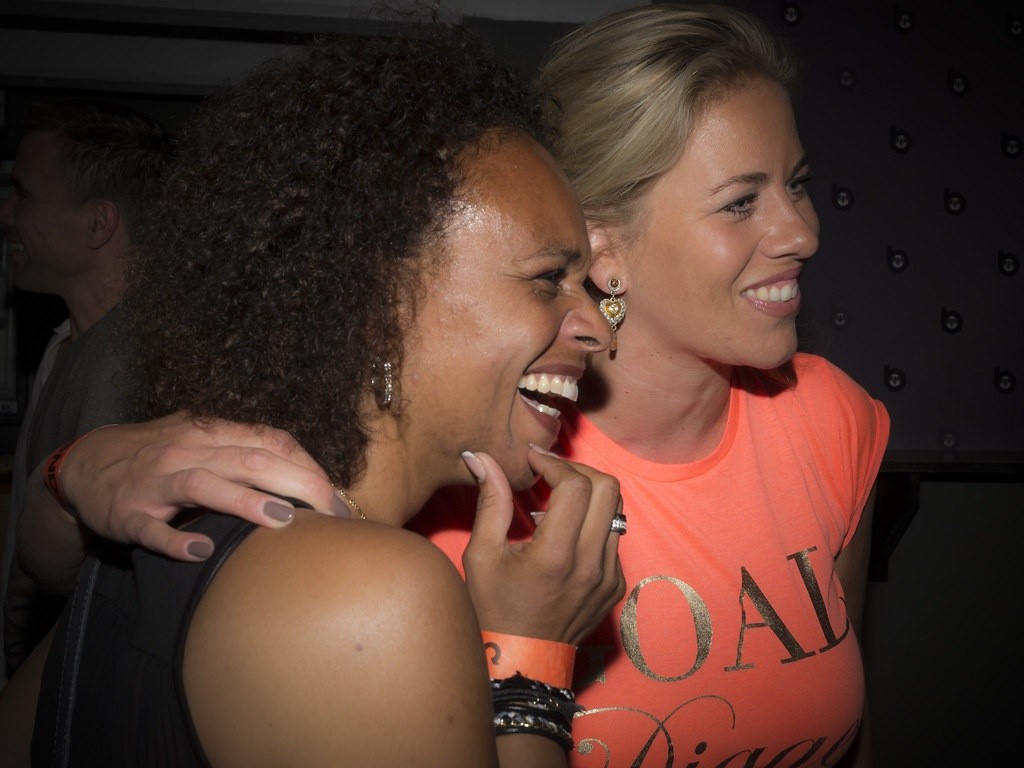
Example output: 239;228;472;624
611;513;628;534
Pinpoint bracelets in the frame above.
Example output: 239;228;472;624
489;675;575;723
478;631;578;689
43;423;122;515
488;711;575;752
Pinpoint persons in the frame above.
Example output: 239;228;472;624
0;3;892;767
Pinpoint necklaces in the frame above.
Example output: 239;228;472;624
334;484;367;521
